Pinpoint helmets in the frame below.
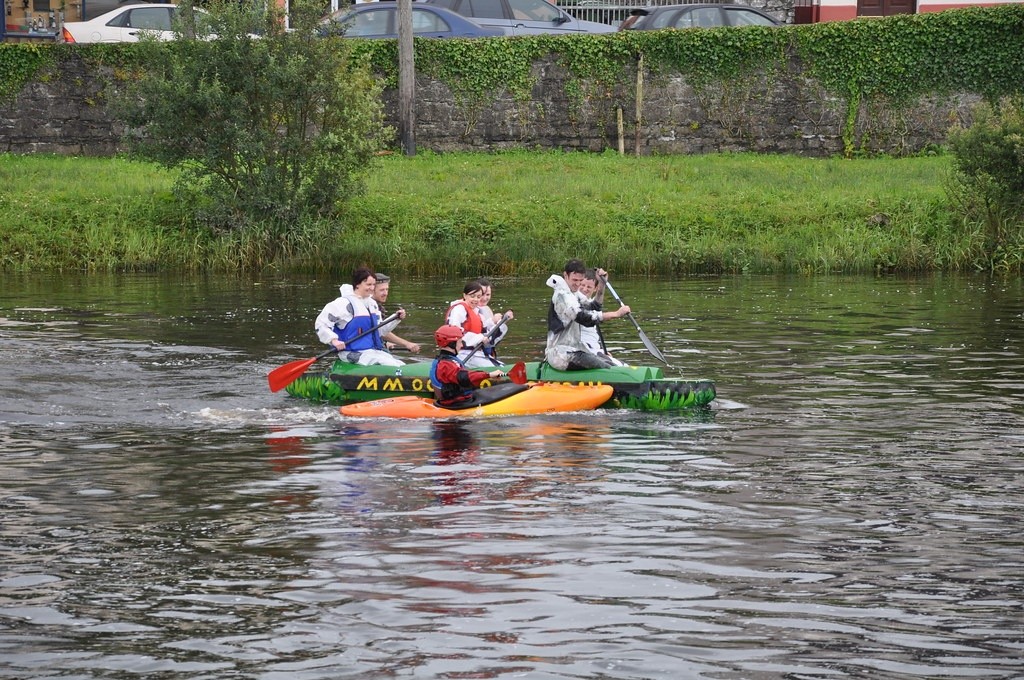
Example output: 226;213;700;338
436;326;463;350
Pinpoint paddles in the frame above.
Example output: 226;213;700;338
389;345;410;352
268;312;401;393
595;320;608;354
497;362;528;384
462;309;514;363
592;267;667;364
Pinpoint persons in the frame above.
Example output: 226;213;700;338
314;267;421;368
444;278;514;369
544;259;633;371
430;325;505;406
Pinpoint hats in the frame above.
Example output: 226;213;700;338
375;272;392;284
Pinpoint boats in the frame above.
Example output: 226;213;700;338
339;381;615;419
287;359;716;412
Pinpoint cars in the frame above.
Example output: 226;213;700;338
619;4;787;33
63;3;263;43
413;0;614;36
309;1;505;38
